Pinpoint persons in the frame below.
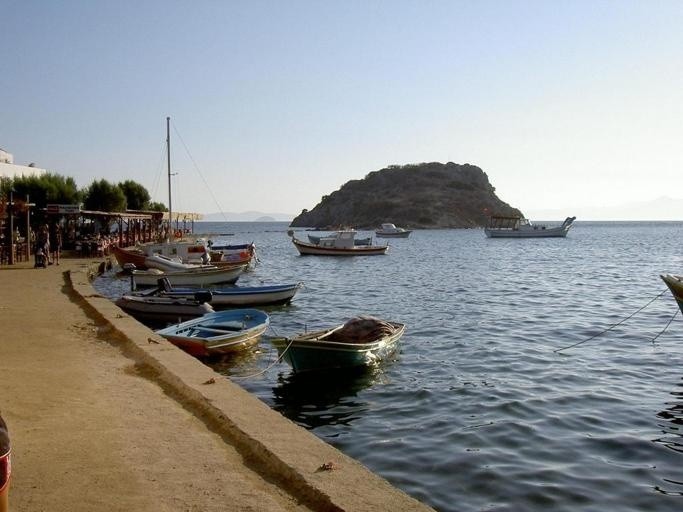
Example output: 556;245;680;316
12;220;182;267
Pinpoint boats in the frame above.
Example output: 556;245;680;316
272;319;406;375
659;273;683;315
482;214;576;238
287;226;390;256
156;307;269;360
305;230;373;245
375;223;412;238
116;295;216;320
107;241;301;314
215;346;270;378
274;372;373;402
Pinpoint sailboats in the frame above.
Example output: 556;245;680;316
144;116;256;266
